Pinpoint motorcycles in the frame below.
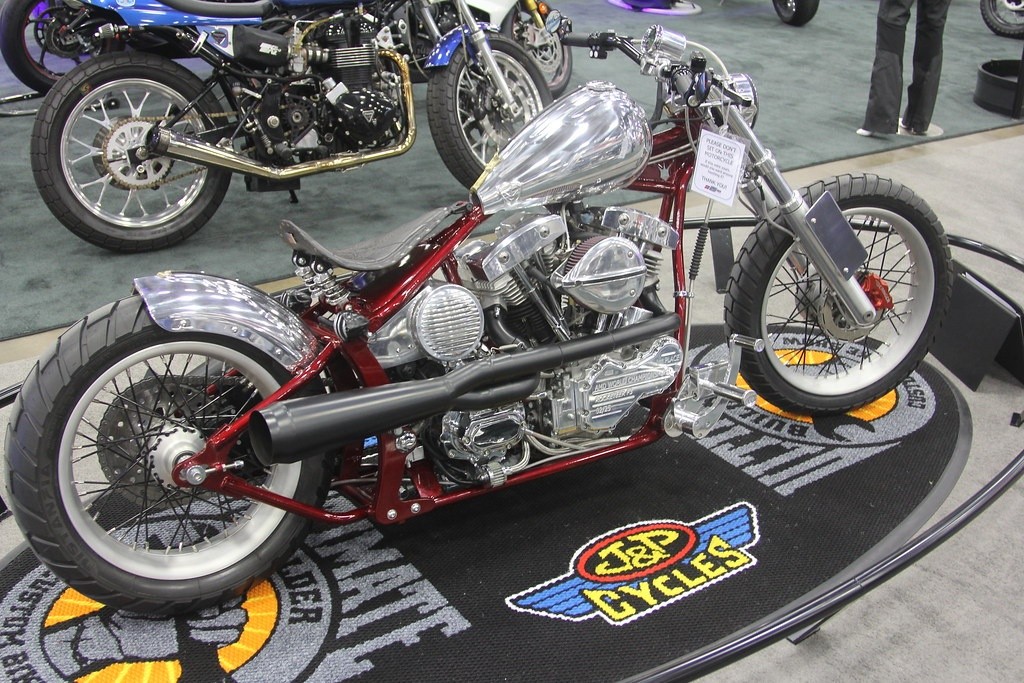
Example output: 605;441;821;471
0;0;589;260
0;30;955;626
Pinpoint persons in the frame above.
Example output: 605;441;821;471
855;0;952;136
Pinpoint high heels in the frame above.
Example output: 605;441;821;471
899;117;916;135
856;128;874;136
